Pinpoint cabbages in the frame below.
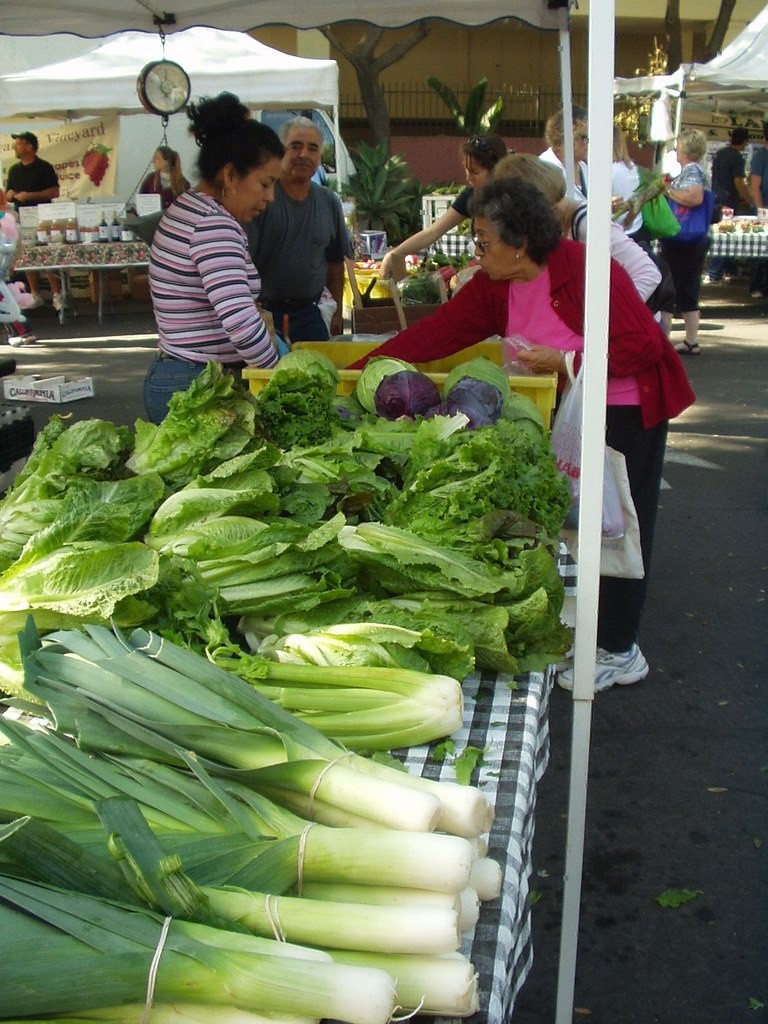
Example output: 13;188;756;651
273;347;545;445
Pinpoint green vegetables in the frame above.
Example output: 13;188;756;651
0;358;576;706
405;252;472;303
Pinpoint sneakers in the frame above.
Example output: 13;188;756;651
27;292;46;309
52;293;69;311
557;642;649;693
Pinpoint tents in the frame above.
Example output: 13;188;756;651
614;2;766;215
0;30;360;205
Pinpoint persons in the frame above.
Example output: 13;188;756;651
127;145;191;218
492;153;662;305
6;132;60;213
702;127;757;287
348;177;695;694
143;93;286;426
538;104;625;214
380;132;516;278
243;115;353;350
750;121;768;209
661;129;712;356
612;126;650;255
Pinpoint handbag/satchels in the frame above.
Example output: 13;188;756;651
637;165;682;238
549;351;645;579
662;165;717;250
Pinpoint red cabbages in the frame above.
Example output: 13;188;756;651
427;389;493;429
374;369;440;421
334;405;360;421
447;376;502;419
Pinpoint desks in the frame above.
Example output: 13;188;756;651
653;215;768;257
348;488;580;1024
15;261;151;325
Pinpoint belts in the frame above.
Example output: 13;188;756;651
160;351;249;368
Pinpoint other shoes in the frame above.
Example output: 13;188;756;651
721;273;739;282
703;275;728;287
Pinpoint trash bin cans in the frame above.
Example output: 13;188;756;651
359;227;388;259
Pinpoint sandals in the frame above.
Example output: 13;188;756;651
674;339;702;355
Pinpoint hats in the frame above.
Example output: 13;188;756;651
11;131;38;152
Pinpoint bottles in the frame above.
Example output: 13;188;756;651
99;211;108;242
36;220;49;242
50;220;61;236
66;218;77;245
111;210;119;242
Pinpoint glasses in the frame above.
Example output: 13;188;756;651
467;133;500;163
472;235;504;253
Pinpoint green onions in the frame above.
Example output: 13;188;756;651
0;613;503;1024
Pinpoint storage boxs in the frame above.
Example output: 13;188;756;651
240;261;561;436
3;371;93;403
